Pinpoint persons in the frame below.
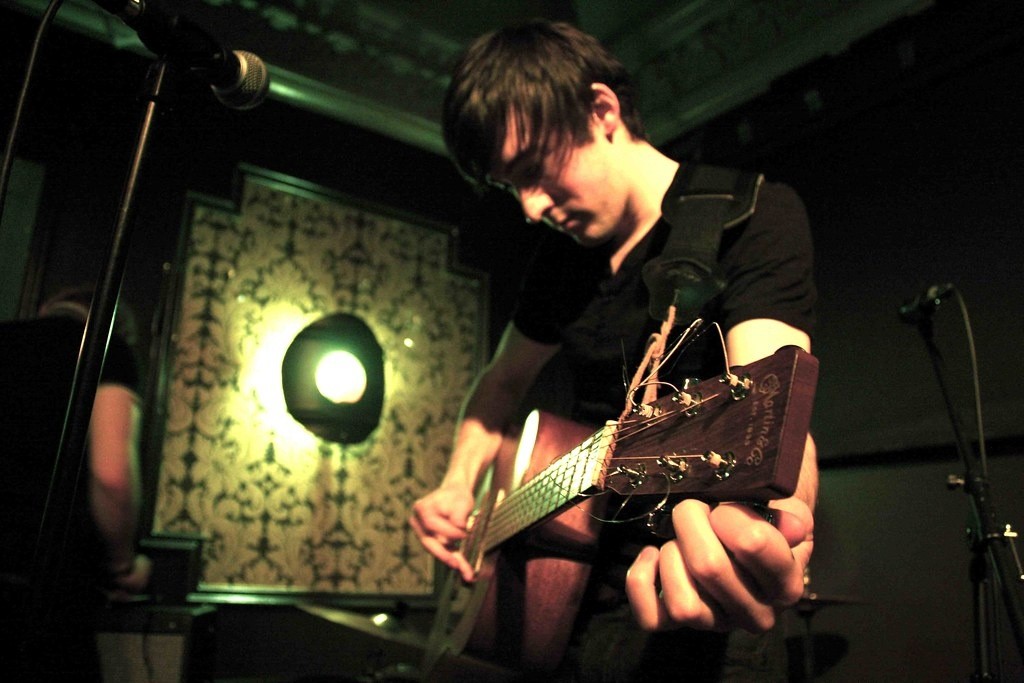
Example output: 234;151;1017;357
406;20;821;683
39;286;154;683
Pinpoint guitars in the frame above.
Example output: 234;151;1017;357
416;341;821;683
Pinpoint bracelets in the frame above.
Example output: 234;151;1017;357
112;558;135;576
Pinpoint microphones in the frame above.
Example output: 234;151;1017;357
94;0;268;109
896;284;954;324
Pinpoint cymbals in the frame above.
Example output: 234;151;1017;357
790;590;873;618
